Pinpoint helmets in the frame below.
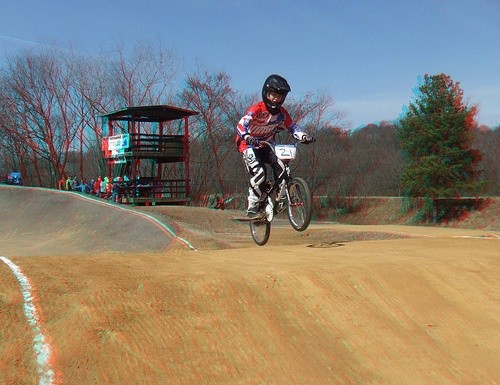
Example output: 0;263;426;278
262;74;291;116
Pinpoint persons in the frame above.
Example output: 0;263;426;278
235;74;316;218
58;175;151;203
214;196;225;210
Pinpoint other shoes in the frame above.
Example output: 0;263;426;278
247;209;258;217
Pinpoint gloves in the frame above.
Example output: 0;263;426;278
245;136;259;146
304;135;315;143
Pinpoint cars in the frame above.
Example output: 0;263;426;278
5;172;23;185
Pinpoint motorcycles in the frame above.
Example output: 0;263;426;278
245;138;312;245
